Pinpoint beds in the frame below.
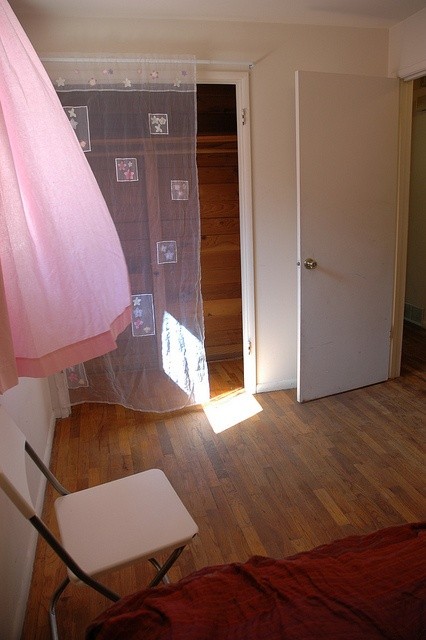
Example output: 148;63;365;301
94;509;426;640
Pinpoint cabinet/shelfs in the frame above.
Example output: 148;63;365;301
77;136;245;379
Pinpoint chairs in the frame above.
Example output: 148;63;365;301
0;402;197;640
